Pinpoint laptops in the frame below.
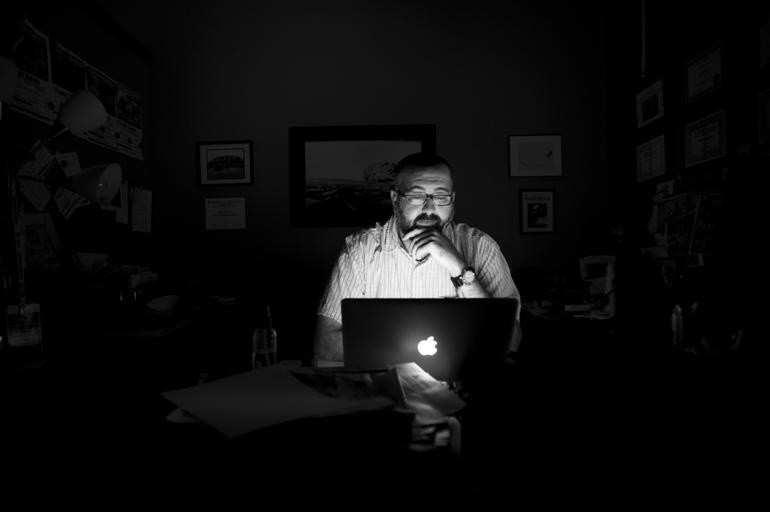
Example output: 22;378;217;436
339;296;519;374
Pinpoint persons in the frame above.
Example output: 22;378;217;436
312;149;526;363
384;360;468;469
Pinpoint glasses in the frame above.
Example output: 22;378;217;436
398;193;454;206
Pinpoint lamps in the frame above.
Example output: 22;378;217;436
7;87;109;352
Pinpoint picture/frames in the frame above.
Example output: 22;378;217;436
195;139;253;187
506;131;563;178
285;123;437;228
520;186;555;234
634;44;728;234
203;194;246;233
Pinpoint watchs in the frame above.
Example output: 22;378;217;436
447;263;476;289
